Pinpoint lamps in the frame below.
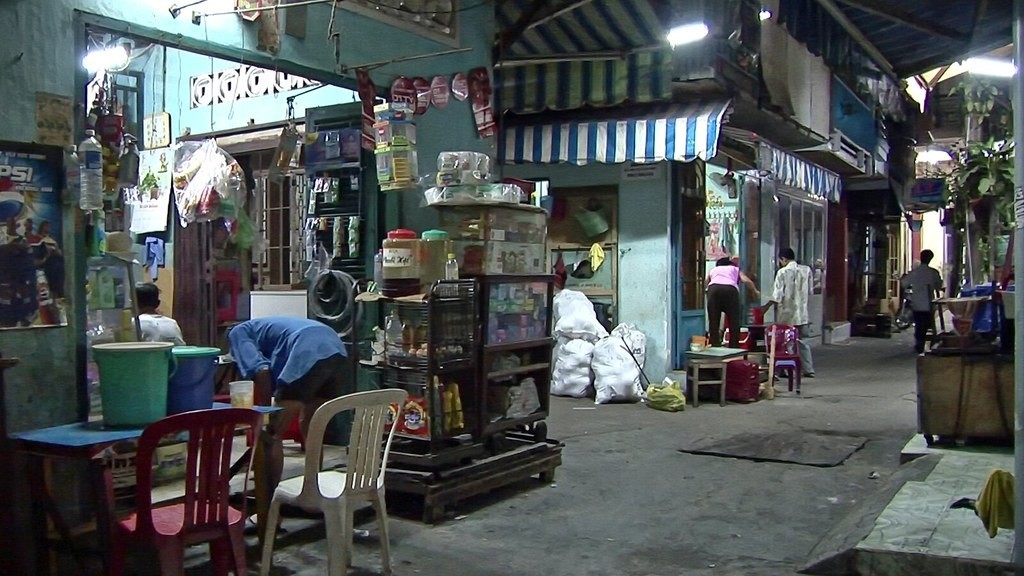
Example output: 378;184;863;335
665;19;713;52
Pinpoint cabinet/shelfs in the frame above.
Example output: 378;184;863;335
299;118;371;263
351;202;557;455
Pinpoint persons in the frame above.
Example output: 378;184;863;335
132;282;184;349
703;258;761;348
901;250;945;353
224;315;349;508
762;246;816;377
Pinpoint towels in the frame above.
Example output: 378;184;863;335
975;467;1017;537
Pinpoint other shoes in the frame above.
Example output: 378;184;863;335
804;372;815;377
780;373;790;378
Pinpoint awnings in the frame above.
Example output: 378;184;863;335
714;126;843;204
498;92;734;167
490;1;674;115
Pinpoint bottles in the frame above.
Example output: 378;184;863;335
401;320;413;357
101;141;140;195
385;309;403;366
65;144;81;193
445;253;459;296
88;266;125;310
77;129;104;211
382;229;421;279
36;269;65;324
372;326;399;355
421;229;454;282
373;248;421;297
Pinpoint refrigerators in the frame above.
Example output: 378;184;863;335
250;289;308;321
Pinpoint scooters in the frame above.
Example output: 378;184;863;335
891;270;918;331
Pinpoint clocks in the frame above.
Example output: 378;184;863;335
142;111;172;150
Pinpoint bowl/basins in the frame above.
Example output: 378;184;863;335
690;343;703;351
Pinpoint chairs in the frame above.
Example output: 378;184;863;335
762;322;801;396
114;403;265;576
259;388;410;576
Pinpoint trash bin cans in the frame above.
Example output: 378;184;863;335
962;285;1016;339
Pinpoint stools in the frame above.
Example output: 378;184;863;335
683;357;727;409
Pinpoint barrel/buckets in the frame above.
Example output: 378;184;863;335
166;345;222;416
91;342;174;430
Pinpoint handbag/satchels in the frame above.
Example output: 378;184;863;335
644;380;688;412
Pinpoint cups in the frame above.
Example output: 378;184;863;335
229;381;255;408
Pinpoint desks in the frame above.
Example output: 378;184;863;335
6;401;290;576
